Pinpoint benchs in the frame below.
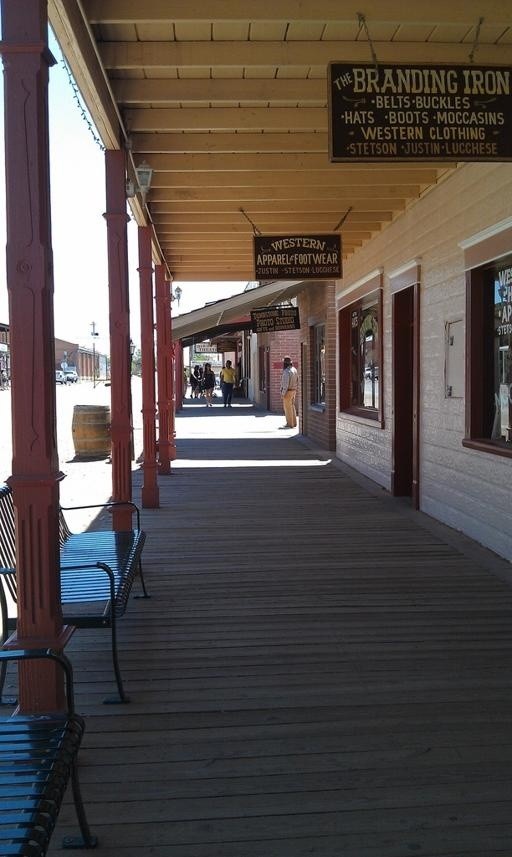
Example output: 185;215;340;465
0;647;96;855
2;481;147;704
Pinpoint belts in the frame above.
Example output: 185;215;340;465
288;389;296;392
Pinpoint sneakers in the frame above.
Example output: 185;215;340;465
278;424;293;430
223;404;233;408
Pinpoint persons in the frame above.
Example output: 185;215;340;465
181;358;237;409
278;354;298;428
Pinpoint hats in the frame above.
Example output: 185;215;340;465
281;356;293;364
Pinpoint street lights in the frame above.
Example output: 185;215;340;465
175;288;182;308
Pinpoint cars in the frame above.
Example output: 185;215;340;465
364;367;379;380
55;370;78;384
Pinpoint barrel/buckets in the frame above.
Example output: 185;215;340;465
71;404;112;459
